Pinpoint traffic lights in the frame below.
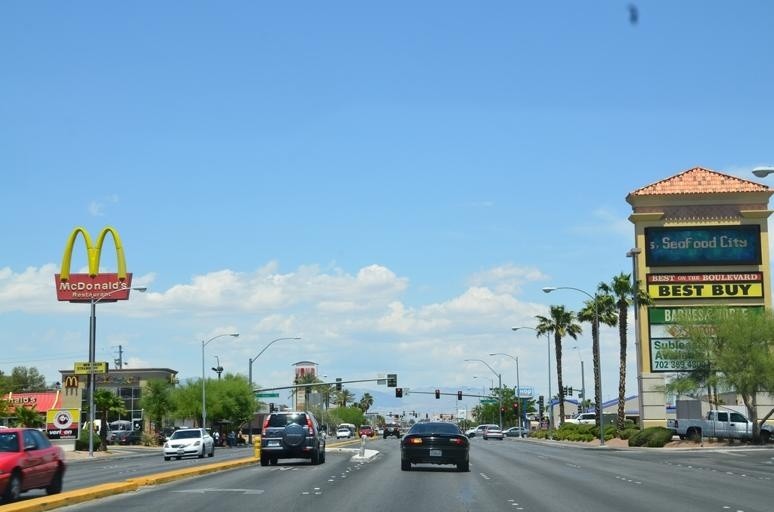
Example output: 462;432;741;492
458;390;462;400
568;386;572;396
396;387;402;397
563;385;567;396
500;407;504;412
513;402;517;409
435;390;439;399
577;405;582;413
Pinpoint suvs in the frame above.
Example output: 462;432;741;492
558;411;596;427
259;409;325;467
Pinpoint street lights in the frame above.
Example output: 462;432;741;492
752;167;774;178
511;325;554;431
472;375;494;395
247;336;302;444
490;352;523;438
463;359;502;431
200;331;239;428
572;346;586;413
88;285;148;455
542;285;607;445
310;374;327;382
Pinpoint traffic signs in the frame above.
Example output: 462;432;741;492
403;388;409;396
255;393;280;397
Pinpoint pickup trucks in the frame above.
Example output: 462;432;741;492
666;409;773;445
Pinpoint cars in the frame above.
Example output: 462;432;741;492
335;422;411;440
0;425;66;502
81;418;142;445
398;420;470;472
162;427;214;461
465;423;529;440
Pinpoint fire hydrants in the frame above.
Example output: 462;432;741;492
253;436;260;458
